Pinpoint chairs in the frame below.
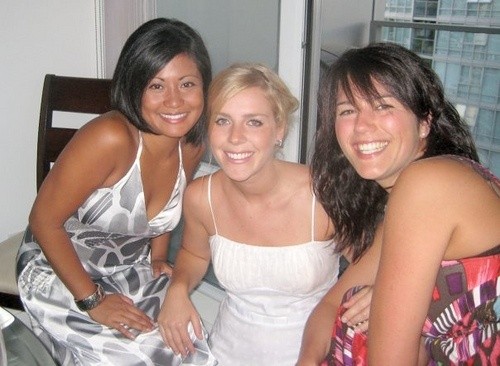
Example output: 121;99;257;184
0;74;117;366
